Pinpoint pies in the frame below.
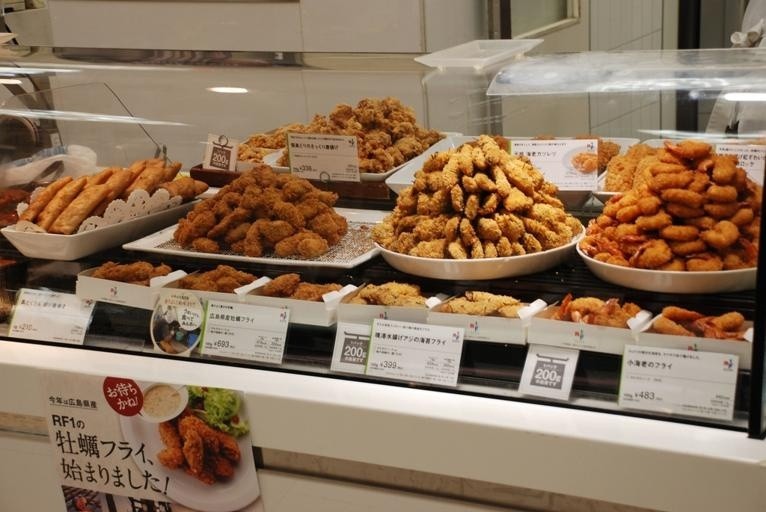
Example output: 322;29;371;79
19;159;208;234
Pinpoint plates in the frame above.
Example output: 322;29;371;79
373;222;586;282
0;198;202;261
385;131;641;211
576;232;759;294
412;37;545;70
122;206;394;270
119;379;261;511
270;131;464;182
591;138;756;208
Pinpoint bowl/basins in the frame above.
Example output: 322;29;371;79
137;382;189;423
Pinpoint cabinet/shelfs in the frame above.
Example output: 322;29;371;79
0;1;766;512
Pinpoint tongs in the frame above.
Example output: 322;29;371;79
22;160;63;191
